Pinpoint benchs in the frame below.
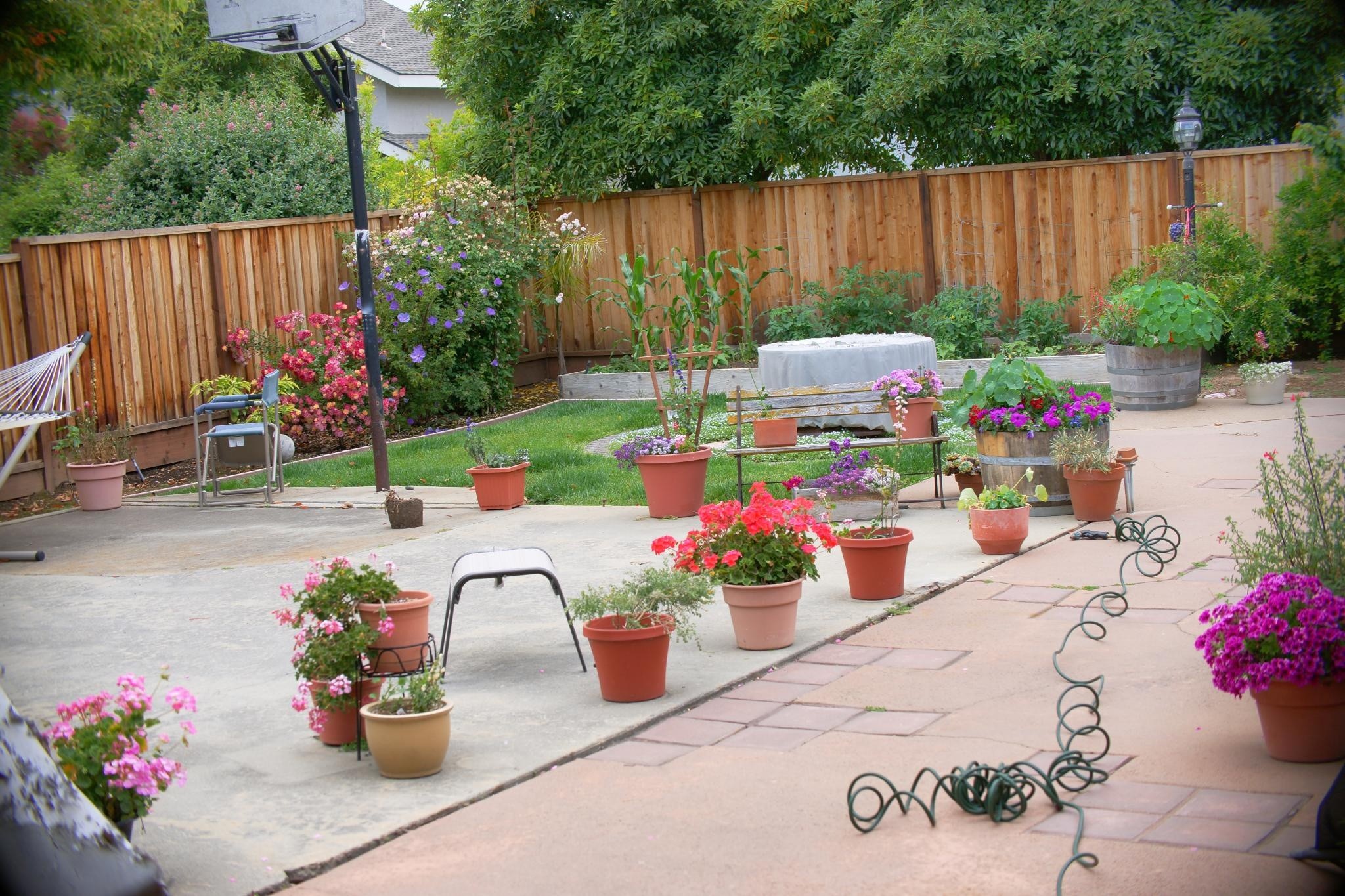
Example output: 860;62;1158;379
726;377;952;509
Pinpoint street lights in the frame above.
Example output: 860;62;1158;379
1163;85;1228;272
204;6;390;490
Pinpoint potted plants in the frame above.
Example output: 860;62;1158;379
563;560;715;703
941;425;1127;556
738;340;798;448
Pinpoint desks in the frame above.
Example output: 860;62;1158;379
756;332;937;433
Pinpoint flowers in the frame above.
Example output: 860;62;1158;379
40;552;446;820
49;357;140;464
1195;572;1345;699
462;346;908;586
871;261;1293;447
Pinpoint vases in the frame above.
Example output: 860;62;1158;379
360;698;455;779
972;422;1111;516
887;396;936;440
1243;373;1287;406
634;448;916;651
1104;343;1203;412
381;462;531;530
114;819;134;841
307;678;386;745
67;460;127;512
355;590;434;673
1247;672;1345;765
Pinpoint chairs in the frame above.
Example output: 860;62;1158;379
193;368;283;510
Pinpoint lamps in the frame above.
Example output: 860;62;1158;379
1114;448;1138;468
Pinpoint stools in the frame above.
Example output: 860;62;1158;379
438;545;589;677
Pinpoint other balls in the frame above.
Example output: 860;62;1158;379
280;433;295;463
